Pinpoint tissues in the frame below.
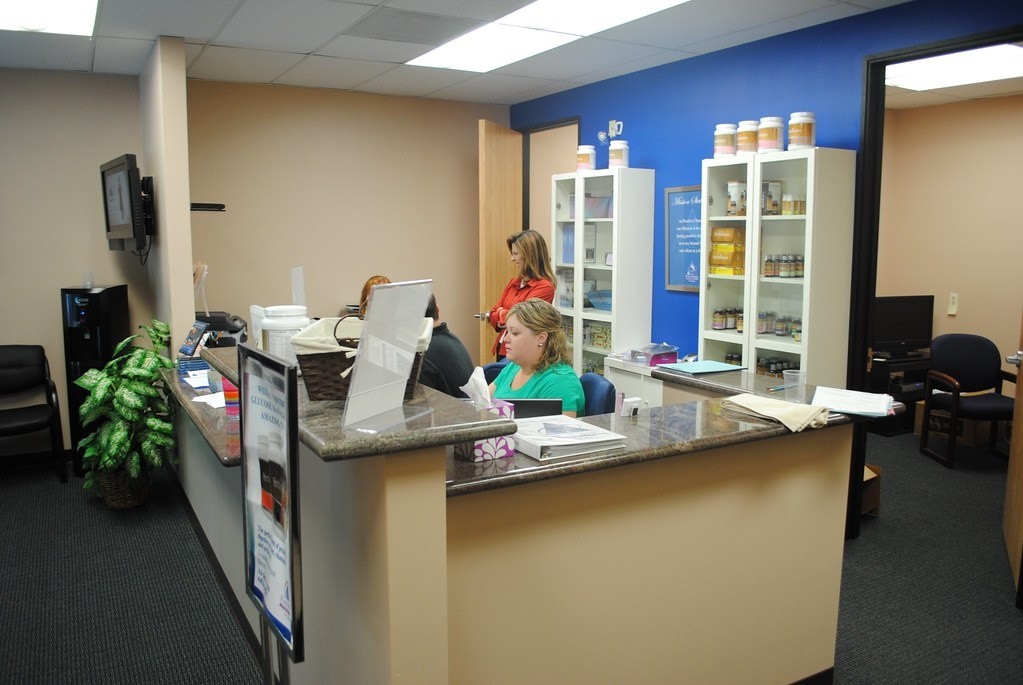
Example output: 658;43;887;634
454;365;515;463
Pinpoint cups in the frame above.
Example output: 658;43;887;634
783;369;806;403
82;271;94;289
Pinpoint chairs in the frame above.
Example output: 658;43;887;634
580;372;616;416
919;333;1017;469
0;344;67;483
481;356;510;385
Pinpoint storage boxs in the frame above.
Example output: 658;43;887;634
914;399;1007;449
864;464;882;520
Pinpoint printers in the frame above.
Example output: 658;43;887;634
196;312;250;348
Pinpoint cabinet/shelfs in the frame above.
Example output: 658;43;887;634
550;167;655;377
697;146;856;389
868;351;930;437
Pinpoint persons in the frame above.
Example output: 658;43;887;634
419;293;474;399
487;298;585;419
358;276;391;320
491;230;557;363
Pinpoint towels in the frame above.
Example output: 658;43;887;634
719;393;830;433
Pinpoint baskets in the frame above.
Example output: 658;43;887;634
95;446;172;508
291;299;434;400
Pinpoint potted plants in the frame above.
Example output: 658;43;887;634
73;319;179;511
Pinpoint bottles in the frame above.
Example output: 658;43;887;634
260;305;310;376
725;179;746;216
757;117;785;153
736;120;759;156
725;353;744;367
764;253;804;278
713;123;738;158
576;145;597;172
756;356;800;377
757;311;801;342
712;306;743;333
762;178;806;215
788;111;816;150
257;432;289;529
608;140;629;169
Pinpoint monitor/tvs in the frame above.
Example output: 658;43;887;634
100;153;147;250
